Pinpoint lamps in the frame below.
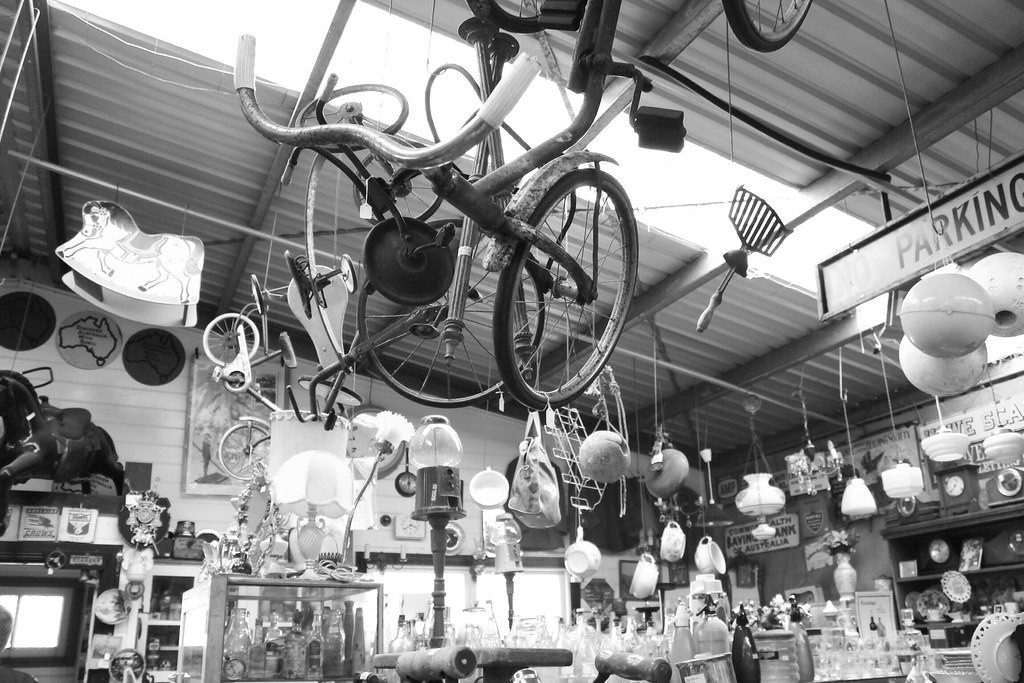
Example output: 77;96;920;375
410;0;1024;648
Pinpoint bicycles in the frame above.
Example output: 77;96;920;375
203;0;816;481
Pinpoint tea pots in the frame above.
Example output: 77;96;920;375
564;525;602;584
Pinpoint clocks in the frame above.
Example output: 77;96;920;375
395;511;428;539
441;522;464;556
342;404;408;478
986;467;1024;508
984;526;1024;566
395;448;417;496
934;464;981;515
930;538;958;573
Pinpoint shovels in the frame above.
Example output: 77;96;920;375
696;185;794;332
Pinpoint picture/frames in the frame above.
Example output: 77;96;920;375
853;591;898;640
617;559;649;601
899;558;917;578
960;533;985;571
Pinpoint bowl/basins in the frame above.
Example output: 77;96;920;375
469;466;509;510
629;552;660;599
694;536;726;577
659;519;686;561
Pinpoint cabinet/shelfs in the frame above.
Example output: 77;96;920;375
881;502;1023;655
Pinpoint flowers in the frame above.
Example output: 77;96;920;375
820;530;861;555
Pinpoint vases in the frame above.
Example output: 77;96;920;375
834;553;858;601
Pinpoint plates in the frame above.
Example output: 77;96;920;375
108;647;147;683
904;591;924;614
917;587;951;621
940;571;972;603
93;587;133;625
118;494;171;547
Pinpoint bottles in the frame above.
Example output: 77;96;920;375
222;599;366;682
385;609;671;683
804;611;930;683
